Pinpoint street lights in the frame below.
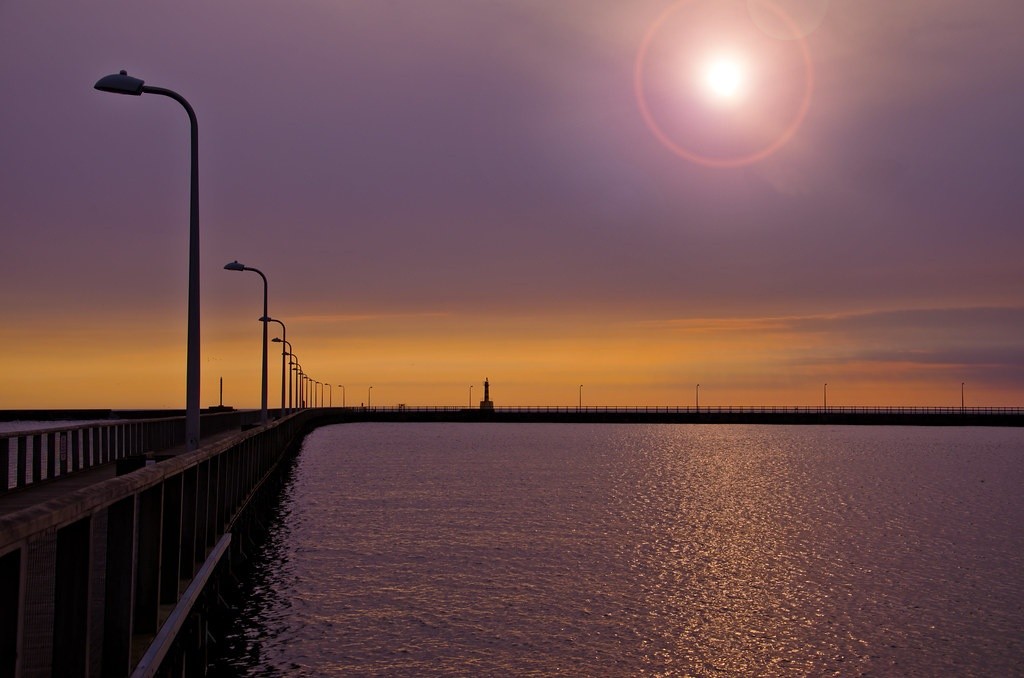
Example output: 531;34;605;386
309;378;317;408
258;316;285;417
224;261;268;423
369;387;372;410
580;385;583;413
696;384;699;413
824;384;827;412
282;352;298;414
316;381;323;407
338;385;345;407
325;383;331;407
94;69;200;448
470;386;473;408
289;361;308;411
962;383;965;415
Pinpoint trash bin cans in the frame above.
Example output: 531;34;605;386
241;424;260;432
116;455;173;475
